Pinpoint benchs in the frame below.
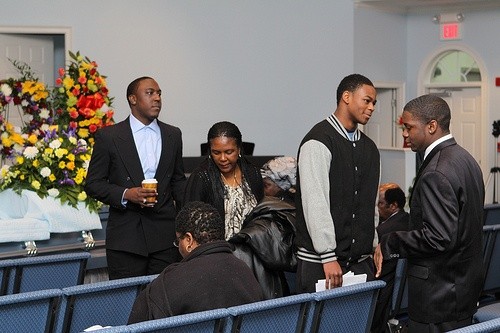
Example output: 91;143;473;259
0;200;500;333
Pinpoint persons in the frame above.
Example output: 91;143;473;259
84;76;188;281
294;73;382;294
127;201;265;324
374;94;485;333
376;183;409;245
183;121;264;241
261;156;298;208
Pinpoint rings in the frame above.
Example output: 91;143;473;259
143;198;146;204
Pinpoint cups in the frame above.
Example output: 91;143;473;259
142;179;158;205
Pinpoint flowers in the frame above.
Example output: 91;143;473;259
0;50;116;214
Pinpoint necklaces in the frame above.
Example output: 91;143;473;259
220;172;237;187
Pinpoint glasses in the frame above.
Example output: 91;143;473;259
173;232;185;247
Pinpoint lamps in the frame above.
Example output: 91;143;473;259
431;10;464;41
